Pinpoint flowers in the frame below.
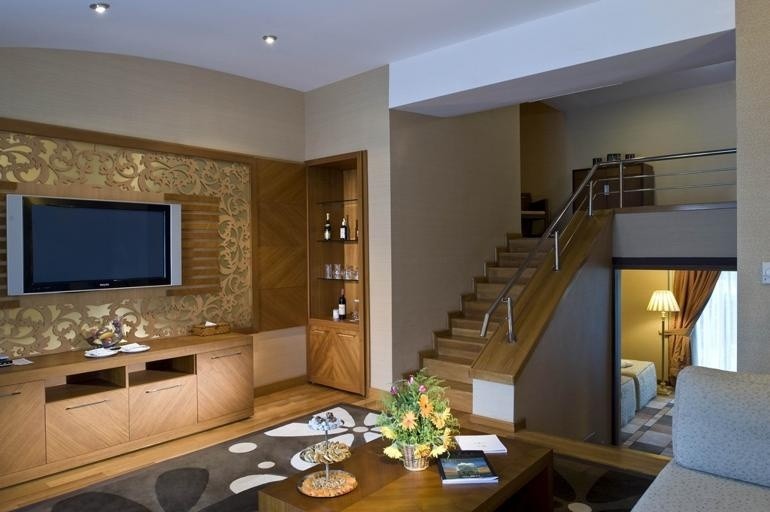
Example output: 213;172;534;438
378;365;461;463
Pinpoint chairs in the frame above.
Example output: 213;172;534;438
522;193;550;235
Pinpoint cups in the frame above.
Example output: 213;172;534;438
353;300;359;320
352;271;358;280
333;263;342;279
592;158;602;166
332;309;340;319
625;154;634;160
323;264;332;279
342;264;351;279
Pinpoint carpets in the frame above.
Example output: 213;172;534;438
6;401;655;511
621;397;675;458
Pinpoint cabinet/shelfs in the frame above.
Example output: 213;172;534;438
1;356;46;490
46;334;198;477
147;328;254;432
572;162;656;214
307;151;369;397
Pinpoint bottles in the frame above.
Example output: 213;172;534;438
354;219;359;239
339;218;348;240
324;213;331;240
338;288;346;320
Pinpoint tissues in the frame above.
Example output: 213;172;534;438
192;320;232;338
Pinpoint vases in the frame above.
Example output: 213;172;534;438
402;441;431;472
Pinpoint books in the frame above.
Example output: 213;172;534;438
437;450;499;486
455;433;509;453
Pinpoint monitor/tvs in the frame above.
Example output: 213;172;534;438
6;193;182;297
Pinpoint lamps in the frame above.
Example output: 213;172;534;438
646;289;682;396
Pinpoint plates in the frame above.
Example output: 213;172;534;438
83;350;118;359
120;345;151;354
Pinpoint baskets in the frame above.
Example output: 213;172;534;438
396;441;430;471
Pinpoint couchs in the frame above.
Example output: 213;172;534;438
621;375;637;427
621;358;658;410
630;365;770;510
